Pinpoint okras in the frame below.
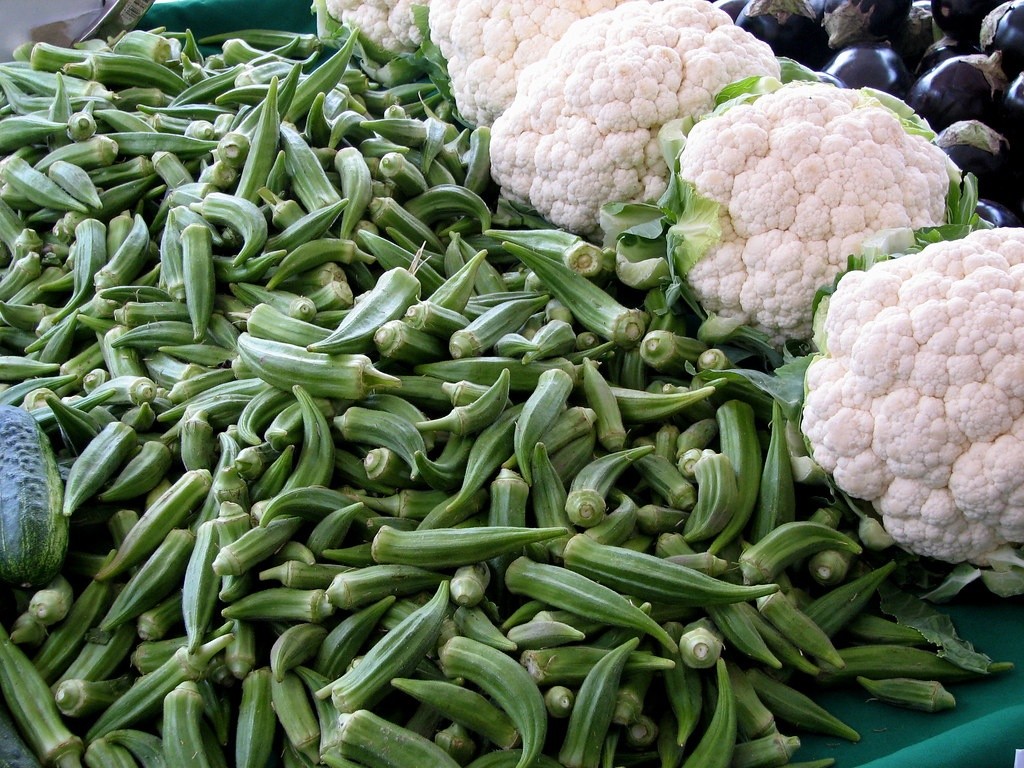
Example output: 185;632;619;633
0;29;1014;768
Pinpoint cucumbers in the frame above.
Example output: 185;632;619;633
0;403;68;589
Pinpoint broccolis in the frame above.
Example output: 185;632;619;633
316;0;1024;571
